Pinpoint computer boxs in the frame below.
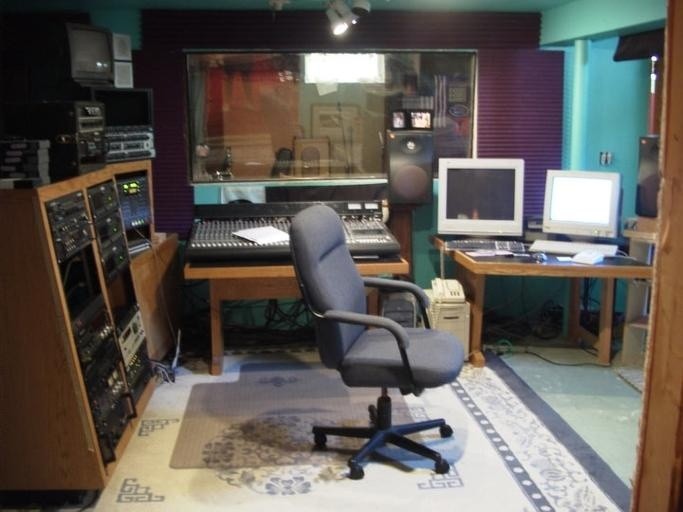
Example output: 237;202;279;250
377;276;417;329
417;288;471;362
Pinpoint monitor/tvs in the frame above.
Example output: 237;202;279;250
437;157;525;239
390;109;409;128
408;108;434;129
542;169;623;244
61;21;114;88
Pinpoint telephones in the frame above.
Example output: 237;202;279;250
431;277;466;303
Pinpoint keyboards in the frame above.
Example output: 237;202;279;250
444;238;526;253
528;239;619;258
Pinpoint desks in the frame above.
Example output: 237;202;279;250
182;257;410;379
427;234;653;371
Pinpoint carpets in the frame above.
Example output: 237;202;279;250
0;338;632;512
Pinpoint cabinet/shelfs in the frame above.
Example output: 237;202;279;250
617;229;660;392
0;161;180;493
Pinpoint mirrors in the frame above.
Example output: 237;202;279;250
181;45;481;186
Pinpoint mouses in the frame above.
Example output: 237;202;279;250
532;252;548;261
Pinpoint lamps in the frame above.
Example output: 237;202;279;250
322;1;374;38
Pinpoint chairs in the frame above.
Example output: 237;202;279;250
288;201;468;478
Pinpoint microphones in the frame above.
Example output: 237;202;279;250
336;102;341;113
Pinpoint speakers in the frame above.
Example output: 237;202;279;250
635;135;660;217
384;129;434;205
